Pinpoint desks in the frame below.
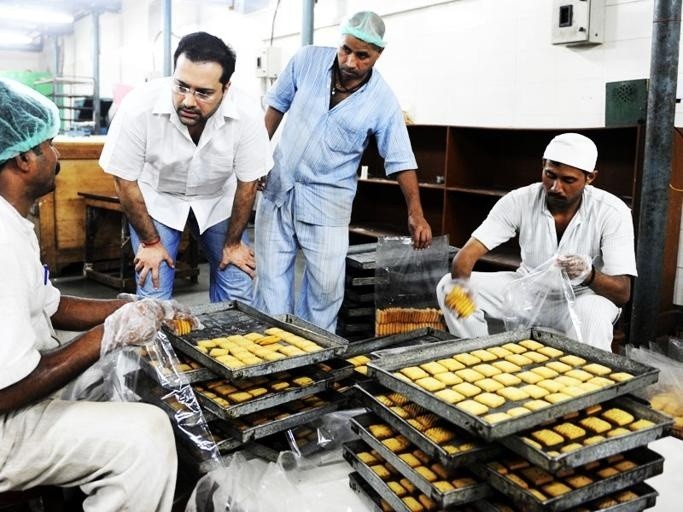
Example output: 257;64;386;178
78;192;200;292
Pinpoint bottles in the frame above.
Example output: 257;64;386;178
292;428;317;450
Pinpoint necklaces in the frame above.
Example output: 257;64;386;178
331;61;373;96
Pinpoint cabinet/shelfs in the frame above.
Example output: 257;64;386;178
349;124;683;340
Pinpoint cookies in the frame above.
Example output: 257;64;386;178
151;385;188;414
521;399;658;457
445;283;478;321
357;448;438;510
203;428;222;447
365;418;475;495
493;452;639;506
270;377;291;393
139;348;201;375
196;379;270;408
649;391;683;433
377;388;475;455
170;314;192;338
390;340;634;427
372;304;447;338
337;352;372;394
293;376;316;389
197;324;325;371
287;395;324;414
232;409;293;434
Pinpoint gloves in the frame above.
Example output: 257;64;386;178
554;249;595;290
442;278;478;322
116;287;206;335
101;295;166;355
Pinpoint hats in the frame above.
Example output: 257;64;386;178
543;131;599;174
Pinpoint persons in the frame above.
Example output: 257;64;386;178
97;32;274;307
435;132;638;353
252;11;432;334
0;77;200;512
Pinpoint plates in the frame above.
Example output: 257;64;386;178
274;313;350;345
178;431;241;462
185;367;337;420
214;387;350;444
162;298;349;383
348;471;389;512
368;342;675;473
366;326;661;442
348;411;487;509
123;357;355;428
123;348;219;386
595;482;660;512
341;439;425;512
478;446;665;512
351;379;653;471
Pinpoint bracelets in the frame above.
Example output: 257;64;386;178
579;264;596;288
140;234;161;248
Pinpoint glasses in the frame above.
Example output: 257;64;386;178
169;83;218;107
